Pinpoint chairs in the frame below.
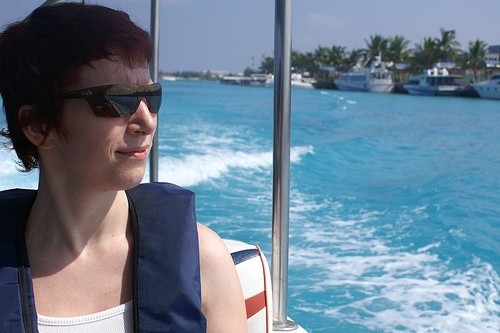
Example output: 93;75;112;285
220;239;273;333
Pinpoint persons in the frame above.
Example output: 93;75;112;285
0;0;247;333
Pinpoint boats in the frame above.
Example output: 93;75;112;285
469;72;500;98
334;66;394;92
403;67;464;95
266;73;314;89
223;74;266;85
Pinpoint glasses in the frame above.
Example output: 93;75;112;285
59;82;163;118
